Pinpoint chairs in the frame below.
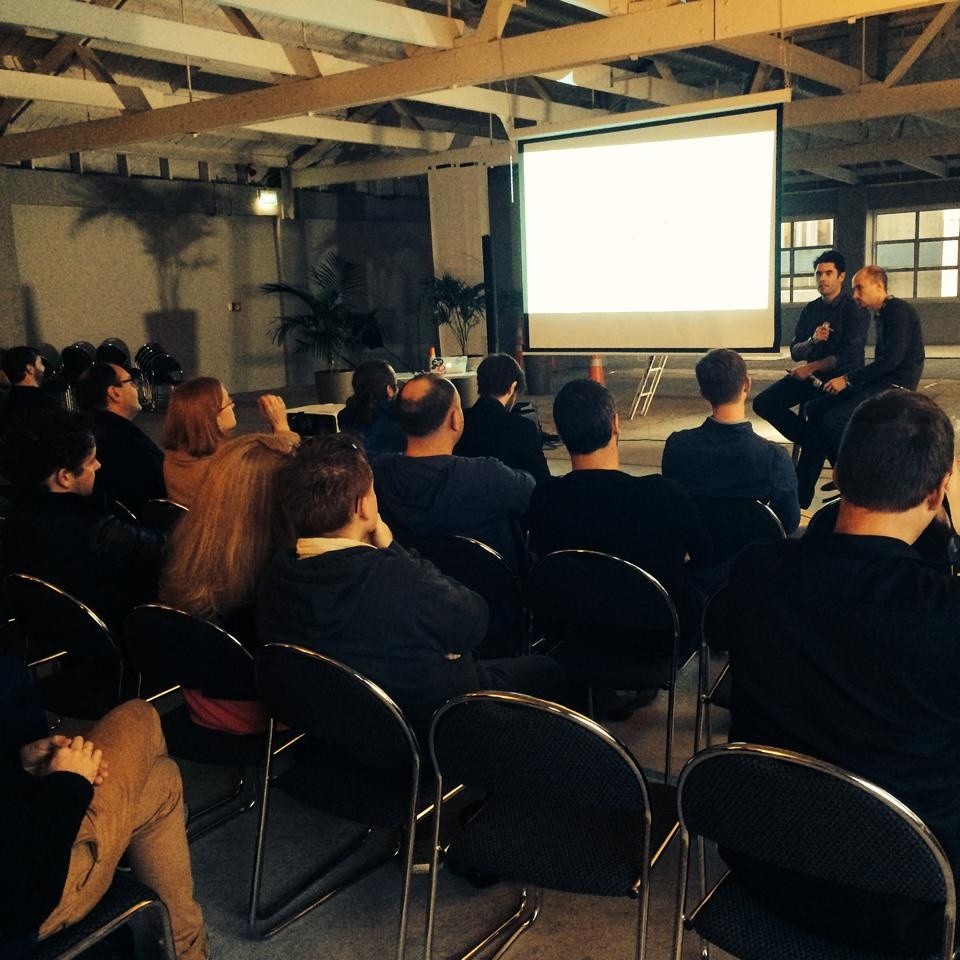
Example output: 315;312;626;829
0;494;959;960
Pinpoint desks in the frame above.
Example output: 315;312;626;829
284;404;346;433
395;372;478;380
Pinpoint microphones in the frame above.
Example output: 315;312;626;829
812;379;824;390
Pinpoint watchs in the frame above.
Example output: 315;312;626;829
844;372;851;388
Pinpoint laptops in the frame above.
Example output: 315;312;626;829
440;355;467;373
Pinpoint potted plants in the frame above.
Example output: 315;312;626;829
261;250;386;404
418;272;489;409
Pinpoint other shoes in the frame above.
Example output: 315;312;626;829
821;480;837;492
801;489;815;509
607;689;657;722
114;801;191;872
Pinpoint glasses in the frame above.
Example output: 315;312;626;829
217;396;237;414
120;376;139;389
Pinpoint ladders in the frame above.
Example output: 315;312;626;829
628;355;668;419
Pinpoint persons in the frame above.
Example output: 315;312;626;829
0;343;960;960
361;372;537;551
523;378;717;649
267;438;552;879
752;251;871;510
806;264;926;490
